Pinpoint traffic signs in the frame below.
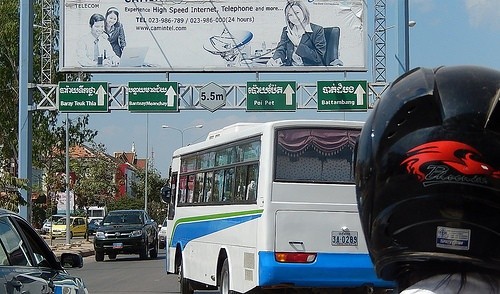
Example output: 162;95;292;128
245;81;297;112
128;81;179;113
57;81;109;113
317;80;368;112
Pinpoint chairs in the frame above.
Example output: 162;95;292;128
181;181;257;203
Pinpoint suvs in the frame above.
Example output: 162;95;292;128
94;209;159;261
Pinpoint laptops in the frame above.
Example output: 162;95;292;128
110;46;149;67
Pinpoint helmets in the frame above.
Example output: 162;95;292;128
355;65;500;280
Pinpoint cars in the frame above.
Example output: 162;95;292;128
43;214;105;240
159;215;168;249
0;208;90;294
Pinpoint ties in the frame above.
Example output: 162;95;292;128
94;40;99;62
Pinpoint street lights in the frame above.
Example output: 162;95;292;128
162;124;203;146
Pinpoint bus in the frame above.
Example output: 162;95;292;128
159;120;399;294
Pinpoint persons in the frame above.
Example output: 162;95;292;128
267;0;326;66
354;64;500;294
75;7;126;67
